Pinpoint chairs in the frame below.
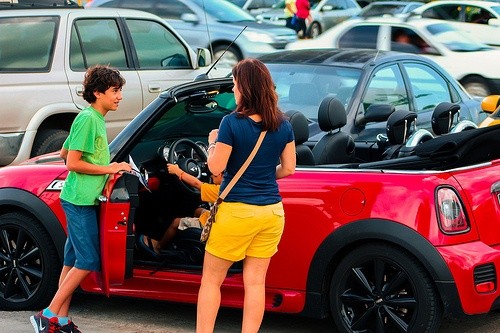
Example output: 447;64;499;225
312;98;356;165
432;103;477;136
285;110;314;166
387;110;433;160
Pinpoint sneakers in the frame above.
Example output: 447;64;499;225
30;310;60;333
60;319;82;333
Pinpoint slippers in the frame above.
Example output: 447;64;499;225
139;234;159;255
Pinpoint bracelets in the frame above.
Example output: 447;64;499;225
179;171;184;181
207;142;215;151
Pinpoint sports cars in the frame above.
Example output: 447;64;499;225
0;77;500;333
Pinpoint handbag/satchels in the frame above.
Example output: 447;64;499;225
200;205;218;241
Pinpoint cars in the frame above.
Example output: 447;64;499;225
0;0;500;167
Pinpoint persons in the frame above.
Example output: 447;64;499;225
195;57;296;333
473;8;493;24
29;65;132;333
395;33;430;54
283;0;312;37
139;163;224;257
